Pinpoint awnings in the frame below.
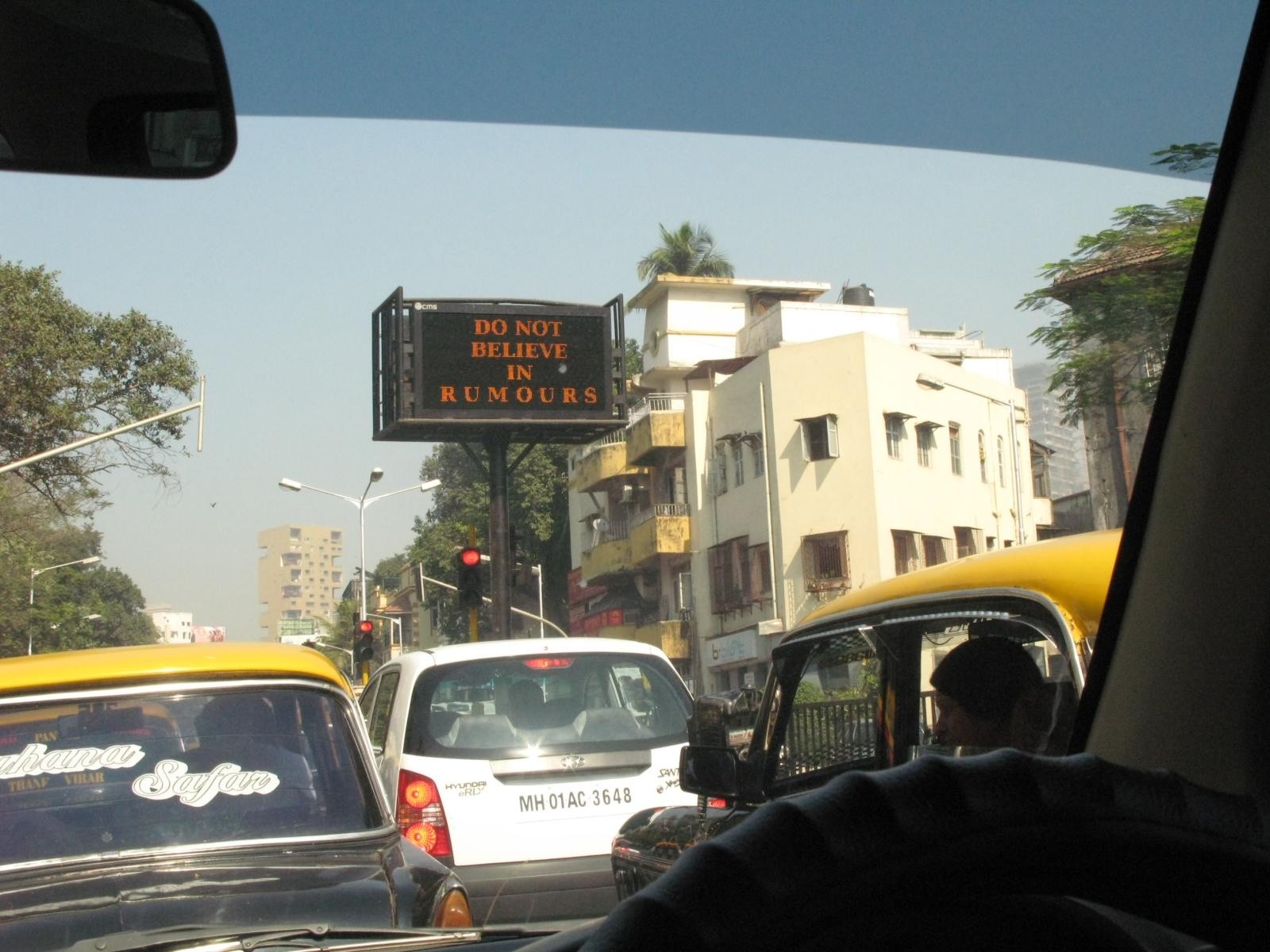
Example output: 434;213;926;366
800;529;849;540
883;412;916;425
1003;529;1016;541
716;432;741;446
981;520;997;539
794;413;838;425
914;420;945;432
921;519;954;540
951;507;984;530
736;432;763;448
682;354;757;395
890;517;925;534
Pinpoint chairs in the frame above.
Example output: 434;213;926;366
535;708;654;747
159;699;317;821
436;714;529;749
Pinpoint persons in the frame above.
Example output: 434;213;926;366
194;694;277;748
929;636;1045;757
508;679;544;729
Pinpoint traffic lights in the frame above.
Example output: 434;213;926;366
356;620;374;661
455;548;483;610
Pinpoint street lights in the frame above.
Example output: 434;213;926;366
913;371;1024;546
274;467;443;689
355;611;403;656
316;641;354;677
27;555;102;656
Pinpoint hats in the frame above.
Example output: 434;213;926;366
929;635;1044;731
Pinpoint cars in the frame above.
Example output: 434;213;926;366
0;643;476;952
355;636;729;928
612;528;1123;901
